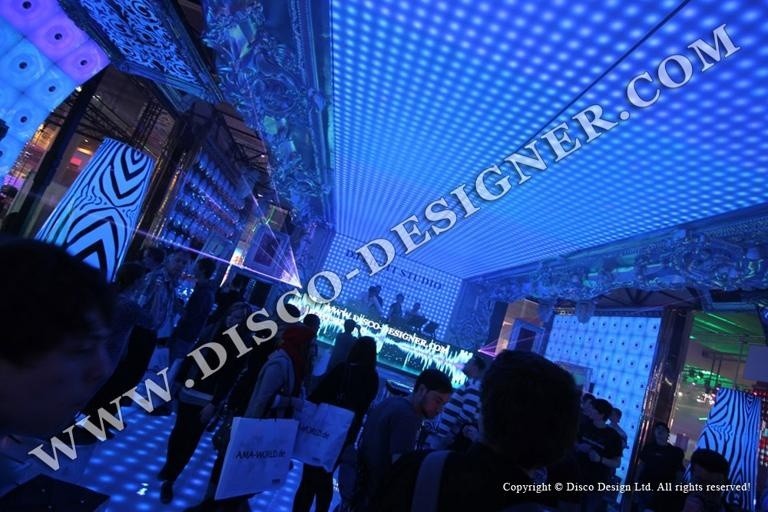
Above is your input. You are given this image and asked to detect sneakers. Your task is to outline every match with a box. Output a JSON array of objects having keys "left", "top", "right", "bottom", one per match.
[{"left": 159, "top": 479, "right": 174, "bottom": 505}]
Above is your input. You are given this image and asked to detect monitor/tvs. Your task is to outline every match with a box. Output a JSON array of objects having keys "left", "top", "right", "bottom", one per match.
[
  {"left": 423, "top": 320, "right": 440, "bottom": 338},
  {"left": 407, "top": 314, "right": 428, "bottom": 333}
]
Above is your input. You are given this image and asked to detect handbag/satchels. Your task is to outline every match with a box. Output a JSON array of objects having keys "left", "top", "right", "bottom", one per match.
[{"left": 213, "top": 359, "right": 301, "bottom": 500}]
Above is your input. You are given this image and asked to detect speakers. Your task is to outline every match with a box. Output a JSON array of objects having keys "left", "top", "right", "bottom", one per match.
[{"left": 265, "top": 206, "right": 288, "bottom": 232}]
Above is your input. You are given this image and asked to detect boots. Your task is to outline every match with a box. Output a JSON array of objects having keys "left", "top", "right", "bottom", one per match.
[{"left": 288, "top": 359, "right": 358, "bottom": 476}]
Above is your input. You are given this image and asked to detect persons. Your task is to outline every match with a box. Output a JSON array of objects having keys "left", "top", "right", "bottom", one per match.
[{"left": 0, "top": 181, "right": 744, "bottom": 512}]
